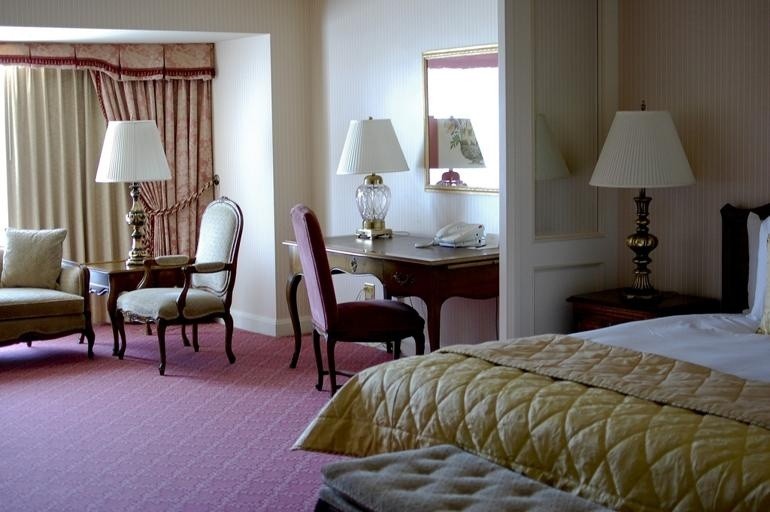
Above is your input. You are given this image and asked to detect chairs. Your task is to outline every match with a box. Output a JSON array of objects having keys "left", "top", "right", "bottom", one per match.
[
  {"left": 116, "top": 197, "right": 243, "bottom": 373},
  {"left": 284, "top": 206, "right": 425, "bottom": 399}
]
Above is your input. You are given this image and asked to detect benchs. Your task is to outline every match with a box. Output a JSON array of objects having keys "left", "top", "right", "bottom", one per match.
[{"left": 310, "top": 440, "right": 609, "bottom": 512}]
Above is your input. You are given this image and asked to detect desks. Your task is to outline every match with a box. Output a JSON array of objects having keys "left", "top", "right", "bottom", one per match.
[{"left": 282, "top": 228, "right": 499, "bottom": 368}]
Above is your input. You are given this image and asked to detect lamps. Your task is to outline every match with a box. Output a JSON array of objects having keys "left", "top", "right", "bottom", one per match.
[
  {"left": 427, "top": 116, "right": 486, "bottom": 188},
  {"left": 336, "top": 116, "right": 409, "bottom": 238},
  {"left": 589, "top": 102, "right": 695, "bottom": 304},
  {"left": 96, "top": 119, "right": 172, "bottom": 265}
]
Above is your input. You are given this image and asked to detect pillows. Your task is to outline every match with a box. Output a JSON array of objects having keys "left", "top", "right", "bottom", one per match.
[
  {"left": 2, "top": 224, "right": 68, "bottom": 291},
  {"left": 745, "top": 212, "right": 760, "bottom": 313},
  {"left": 746, "top": 217, "right": 770, "bottom": 323},
  {"left": 757, "top": 237, "right": 770, "bottom": 334}
]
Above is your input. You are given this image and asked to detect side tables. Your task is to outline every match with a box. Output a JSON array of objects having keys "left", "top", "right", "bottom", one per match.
[{"left": 76, "top": 255, "right": 198, "bottom": 355}]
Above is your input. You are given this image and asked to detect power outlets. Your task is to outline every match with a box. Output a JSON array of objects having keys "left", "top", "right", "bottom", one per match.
[{"left": 362, "top": 282, "right": 376, "bottom": 300}]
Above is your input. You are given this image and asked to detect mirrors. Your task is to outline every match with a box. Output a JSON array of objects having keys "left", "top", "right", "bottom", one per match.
[{"left": 421, "top": 43, "right": 501, "bottom": 194}]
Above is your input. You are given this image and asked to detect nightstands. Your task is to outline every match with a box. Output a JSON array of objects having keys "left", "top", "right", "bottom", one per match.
[{"left": 566, "top": 286, "right": 720, "bottom": 336}]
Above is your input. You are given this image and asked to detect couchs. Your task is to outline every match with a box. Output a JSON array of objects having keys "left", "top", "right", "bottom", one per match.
[{"left": 0, "top": 247, "right": 95, "bottom": 360}]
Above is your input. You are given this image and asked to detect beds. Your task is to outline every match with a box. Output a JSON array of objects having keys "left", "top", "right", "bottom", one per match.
[{"left": 290, "top": 203, "right": 770, "bottom": 512}]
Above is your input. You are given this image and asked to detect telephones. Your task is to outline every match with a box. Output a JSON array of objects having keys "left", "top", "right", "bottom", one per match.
[{"left": 414, "top": 218, "right": 489, "bottom": 252}]
